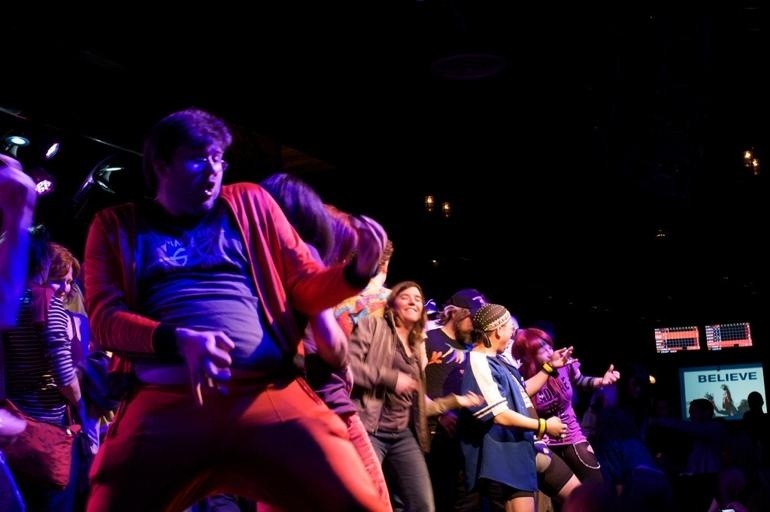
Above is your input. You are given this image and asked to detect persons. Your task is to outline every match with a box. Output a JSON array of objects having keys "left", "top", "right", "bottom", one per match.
[
  {"left": 511, "top": 325, "right": 619, "bottom": 511},
  {"left": 452, "top": 302, "right": 584, "bottom": 512},
  {"left": 671, "top": 399, "right": 726, "bottom": 459},
  {"left": 0, "top": 154, "right": 35, "bottom": 512},
  {"left": 59, "top": 276, "right": 91, "bottom": 383},
  {"left": 744, "top": 392, "right": 769, "bottom": 446},
  {"left": 336, "top": 240, "right": 398, "bottom": 338},
  {"left": 607, "top": 423, "right": 673, "bottom": 497},
  {"left": 345, "top": 280, "right": 483, "bottom": 512},
  {"left": 78, "top": 108, "right": 392, "bottom": 512},
  {"left": 295, "top": 207, "right": 394, "bottom": 512},
  {"left": 422, "top": 290, "right": 489, "bottom": 512},
  {"left": 257, "top": 169, "right": 337, "bottom": 272},
  {"left": 47, "top": 239, "right": 85, "bottom": 369}
]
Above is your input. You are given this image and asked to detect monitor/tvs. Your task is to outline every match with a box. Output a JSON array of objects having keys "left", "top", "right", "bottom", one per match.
[
  {"left": 705, "top": 322, "right": 753, "bottom": 351},
  {"left": 654, "top": 325, "right": 700, "bottom": 356},
  {"left": 681, "top": 364, "right": 770, "bottom": 424}
]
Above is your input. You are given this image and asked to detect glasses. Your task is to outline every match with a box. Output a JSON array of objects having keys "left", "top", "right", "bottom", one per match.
[{"left": 180, "top": 155, "right": 229, "bottom": 174}]
